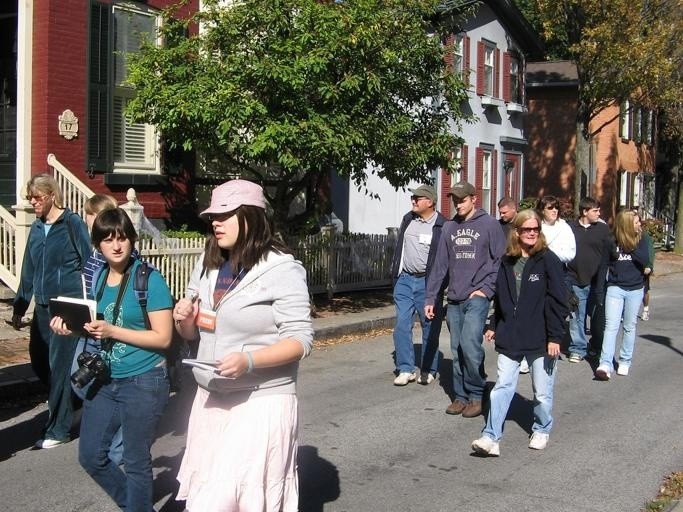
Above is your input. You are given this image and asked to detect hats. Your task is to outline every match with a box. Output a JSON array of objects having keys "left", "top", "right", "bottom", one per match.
[
  {"left": 198, "top": 179, "right": 268, "bottom": 216},
  {"left": 446, "top": 181, "right": 476, "bottom": 199},
  {"left": 407, "top": 184, "right": 437, "bottom": 202}
]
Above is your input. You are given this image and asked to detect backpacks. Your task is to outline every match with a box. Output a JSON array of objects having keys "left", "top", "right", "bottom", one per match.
[{"left": 91, "top": 262, "right": 191, "bottom": 400}]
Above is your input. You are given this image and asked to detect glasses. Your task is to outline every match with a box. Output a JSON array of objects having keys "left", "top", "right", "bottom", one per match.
[
  {"left": 25, "top": 195, "right": 51, "bottom": 201},
  {"left": 544, "top": 206, "right": 560, "bottom": 210},
  {"left": 516, "top": 226, "right": 540, "bottom": 235}
]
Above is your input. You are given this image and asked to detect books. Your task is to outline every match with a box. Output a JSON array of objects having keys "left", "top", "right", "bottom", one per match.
[{"left": 50, "top": 297, "right": 96, "bottom": 333}]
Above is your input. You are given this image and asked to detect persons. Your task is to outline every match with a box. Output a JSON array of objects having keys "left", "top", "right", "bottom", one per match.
[
  {"left": 568, "top": 197, "right": 654, "bottom": 380},
  {"left": 423, "top": 182, "right": 506, "bottom": 417},
  {"left": 171, "top": 181, "right": 314, "bottom": 511},
  {"left": 48, "top": 208, "right": 173, "bottom": 511},
  {"left": 494, "top": 197, "right": 518, "bottom": 241},
  {"left": 519, "top": 195, "right": 577, "bottom": 373},
  {"left": 11, "top": 174, "right": 93, "bottom": 449},
  {"left": 389, "top": 184, "right": 449, "bottom": 384},
  {"left": 470, "top": 208, "right": 567, "bottom": 455},
  {"left": 71, "top": 192, "right": 139, "bottom": 466}
]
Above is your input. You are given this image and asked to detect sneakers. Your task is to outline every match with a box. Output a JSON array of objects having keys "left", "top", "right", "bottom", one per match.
[
  {"left": 471, "top": 435, "right": 500, "bottom": 457},
  {"left": 569, "top": 352, "right": 582, "bottom": 363},
  {"left": 528, "top": 431, "right": 550, "bottom": 450},
  {"left": 519, "top": 359, "right": 530, "bottom": 374},
  {"left": 462, "top": 395, "right": 489, "bottom": 418},
  {"left": 446, "top": 396, "right": 466, "bottom": 415},
  {"left": 394, "top": 370, "right": 417, "bottom": 386},
  {"left": 595, "top": 364, "right": 611, "bottom": 379},
  {"left": 640, "top": 310, "right": 650, "bottom": 321},
  {"left": 417, "top": 371, "right": 440, "bottom": 384},
  {"left": 616, "top": 363, "right": 629, "bottom": 376},
  {"left": 35, "top": 435, "right": 70, "bottom": 449}
]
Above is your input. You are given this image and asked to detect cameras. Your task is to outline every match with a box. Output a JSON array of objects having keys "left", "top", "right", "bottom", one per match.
[{"left": 70, "top": 351, "right": 112, "bottom": 390}]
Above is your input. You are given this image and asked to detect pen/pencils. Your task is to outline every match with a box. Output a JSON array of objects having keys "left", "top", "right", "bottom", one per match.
[{"left": 176, "top": 293, "right": 199, "bottom": 325}]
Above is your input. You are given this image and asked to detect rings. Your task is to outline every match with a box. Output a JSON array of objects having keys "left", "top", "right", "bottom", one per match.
[
  {"left": 554, "top": 352, "right": 557, "bottom": 354},
  {"left": 174, "top": 306, "right": 180, "bottom": 313}
]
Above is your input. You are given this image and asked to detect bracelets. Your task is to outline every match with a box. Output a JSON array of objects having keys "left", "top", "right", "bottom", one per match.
[{"left": 244, "top": 350, "right": 254, "bottom": 375}]
